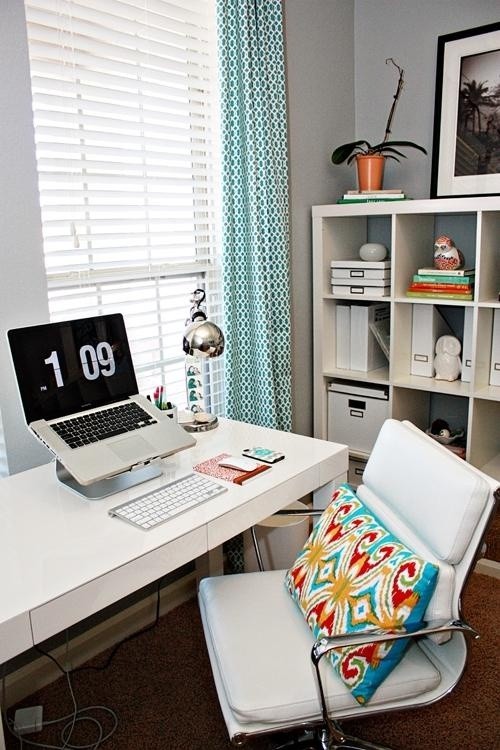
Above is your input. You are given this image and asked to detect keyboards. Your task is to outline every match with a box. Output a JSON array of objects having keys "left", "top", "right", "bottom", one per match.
[{"left": 106, "top": 472, "right": 228, "bottom": 531}]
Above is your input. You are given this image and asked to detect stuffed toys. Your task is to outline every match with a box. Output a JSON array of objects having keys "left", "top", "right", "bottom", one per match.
[
  {"left": 433, "top": 236, "right": 465, "bottom": 269},
  {"left": 434, "top": 334, "right": 462, "bottom": 381},
  {"left": 431, "top": 418, "right": 452, "bottom": 438}
]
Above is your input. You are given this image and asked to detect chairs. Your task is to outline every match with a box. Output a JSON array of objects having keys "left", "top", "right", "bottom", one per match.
[{"left": 196, "top": 418, "right": 500, "bottom": 750}]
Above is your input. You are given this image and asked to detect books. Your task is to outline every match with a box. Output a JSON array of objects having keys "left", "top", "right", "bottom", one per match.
[
  {"left": 337, "top": 189, "right": 415, "bottom": 204},
  {"left": 192, "top": 453, "right": 272, "bottom": 486},
  {"left": 405, "top": 267, "right": 475, "bottom": 302}
]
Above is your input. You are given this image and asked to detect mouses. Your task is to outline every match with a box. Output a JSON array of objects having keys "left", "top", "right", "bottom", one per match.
[{"left": 217, "top": 455, "right": 260, "bottom": 472}]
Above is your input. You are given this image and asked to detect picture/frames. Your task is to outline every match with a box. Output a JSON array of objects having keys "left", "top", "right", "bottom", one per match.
[{"left": 430, "top": 21, "right": 500, "bottom": 199}]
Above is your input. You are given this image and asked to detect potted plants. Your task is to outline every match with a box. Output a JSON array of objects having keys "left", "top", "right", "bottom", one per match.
[{"left": 331, "top": 139, "right": 428, "bottom": 194}]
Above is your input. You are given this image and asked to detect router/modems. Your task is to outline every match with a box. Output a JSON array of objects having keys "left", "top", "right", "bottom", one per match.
[{"left": 15, "top": 706, "right": 44, "bottom": 738}]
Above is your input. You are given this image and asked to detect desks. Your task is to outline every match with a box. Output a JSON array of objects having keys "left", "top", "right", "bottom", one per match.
[{"left": 0, "top": 409, "right": 350, "bottom": 666}]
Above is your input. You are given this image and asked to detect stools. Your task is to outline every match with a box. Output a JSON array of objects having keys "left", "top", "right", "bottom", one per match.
[{"left": 243, "top": 500, "right": 310, "bottom": 574}]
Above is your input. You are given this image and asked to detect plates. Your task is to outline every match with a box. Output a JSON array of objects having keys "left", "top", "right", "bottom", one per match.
[{"left": 426, "top": 426, "right": 464, "bottom": 445}]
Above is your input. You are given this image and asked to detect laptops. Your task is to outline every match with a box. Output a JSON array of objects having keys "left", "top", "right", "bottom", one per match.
[{"left": 6, "top": 312, "right": 197, "bottom": 485}]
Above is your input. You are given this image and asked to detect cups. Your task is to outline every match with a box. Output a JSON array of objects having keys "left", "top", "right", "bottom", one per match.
[{"left": 160, "top": 403, "right": 178, "bottom": 424}]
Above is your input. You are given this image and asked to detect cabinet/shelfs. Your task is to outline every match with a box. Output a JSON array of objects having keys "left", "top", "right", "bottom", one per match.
[{"left": 311, "top": 196, "right": 500, "bottom": 580}]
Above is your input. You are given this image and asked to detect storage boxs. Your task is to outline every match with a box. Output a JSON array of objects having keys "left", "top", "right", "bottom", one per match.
[{"left": 326, "top": 380, "right": 390, "bottom": 454}]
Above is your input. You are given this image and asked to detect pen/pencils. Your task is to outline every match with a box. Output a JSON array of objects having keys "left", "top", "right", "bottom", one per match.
[{"left": 153, "top": 385, "right": 163, "bottom": 411}]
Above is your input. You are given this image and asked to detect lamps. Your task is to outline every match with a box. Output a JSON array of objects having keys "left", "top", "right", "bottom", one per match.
[{"left": 179, "top": 287, "right": 226, "bottom": 433}]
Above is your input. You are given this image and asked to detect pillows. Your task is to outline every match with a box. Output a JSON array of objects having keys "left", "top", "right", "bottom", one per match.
[{"left": 282, "top": 486, "right": 441, "bottom": 707}]
